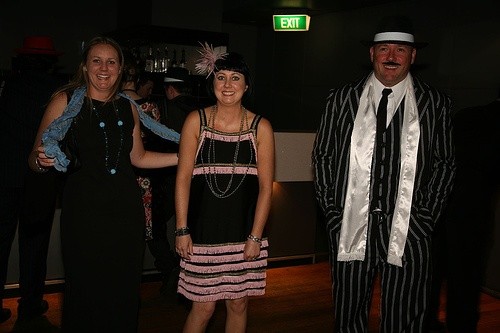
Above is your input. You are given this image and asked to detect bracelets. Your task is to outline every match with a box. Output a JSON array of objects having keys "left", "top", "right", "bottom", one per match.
[
  {"left": 175, "top": 228, "right": 190, "bottom": 236},
  {"left": 35, "top": 160, "right": 45, "bottom": 172},
  {"left": 249, "top": 234, "right": 261, "bottom": 242}
]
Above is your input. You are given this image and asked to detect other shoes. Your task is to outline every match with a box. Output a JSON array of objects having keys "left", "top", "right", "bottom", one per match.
[
  {"left": 0, "top": 309, "right": 10, "bottom": 323},
  {"left": 18, "top": 300, "right": 48, "bottom": 320}
]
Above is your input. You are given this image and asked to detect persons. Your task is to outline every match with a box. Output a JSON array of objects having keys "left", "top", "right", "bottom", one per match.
[
  {"left": 29, "top": 37, "right": 179, "bottom": 333},
  {"left": 173, "top": 53, "right": 275, "bottom": 333},
  {"left": 423, "top": 90, "right": 500, "bottom": 332},
  {"left": 0, "top": 52, "right": 59, "bottom": 321},
  {"left": 312, "top": 24, "right": 455, "bottom": 333},
  {"left": 119, "top": 51, "right": 201, "bottom": 293}
]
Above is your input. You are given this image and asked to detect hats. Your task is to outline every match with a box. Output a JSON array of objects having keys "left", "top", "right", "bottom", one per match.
[
  {"left": 371, "top": 16, "right": 416, "bottom": 48},
  {"left": 160, "top": 67, "right": 192, "bottom": 87},
  {"left": 18, "top": 35, "right": 64, "bottom": 56}
]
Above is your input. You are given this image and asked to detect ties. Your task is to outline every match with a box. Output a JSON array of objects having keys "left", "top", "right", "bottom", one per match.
[{"left": 376, "top": 88, "right": 392, "bottom": 143}]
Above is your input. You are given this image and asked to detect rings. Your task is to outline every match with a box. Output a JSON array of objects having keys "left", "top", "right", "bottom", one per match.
[
  {"left": 179, "top": 253, "right": 181, "bottom": 255},
  {"left": 253, "top": 258, "right": 256, "bottom": 260}
]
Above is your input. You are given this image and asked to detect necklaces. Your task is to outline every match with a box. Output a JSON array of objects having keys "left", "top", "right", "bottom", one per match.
[
  {"left": 201, "top": 104, "right": 252, "bottom": 198},
  {"left": 92, "top": 99, "right": 124, "bottom": 174}
]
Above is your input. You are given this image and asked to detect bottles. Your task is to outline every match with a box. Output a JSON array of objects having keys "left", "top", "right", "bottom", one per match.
[{"left": 132, "top": 45, "right": 187, "bottom": 75}]
